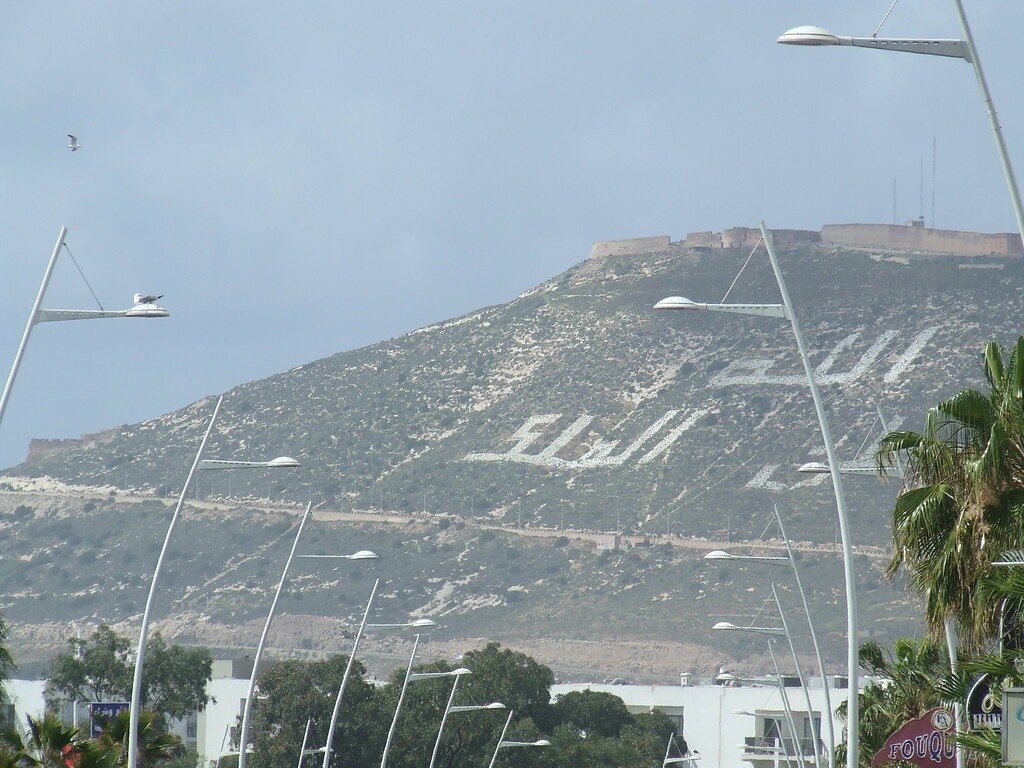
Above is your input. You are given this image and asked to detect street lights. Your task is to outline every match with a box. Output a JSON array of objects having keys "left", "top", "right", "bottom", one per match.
[
  {"left": 777, "top": 3, "right": 1022, "bottom": 252},
  {"left": 799, "top": 397, "right": 969, "bottom": 768},
  {"left": 2, "top": 224, "right": 172, "bottom": 425},
  {"left": 236, "top": 500, "right": 551, "bottom": 767},
  {"left": 650, "top": 222, "right": 858, "bottom": 768},
  {"left": 123, "top": 397, "right": 303, "bottom": 768}
]
